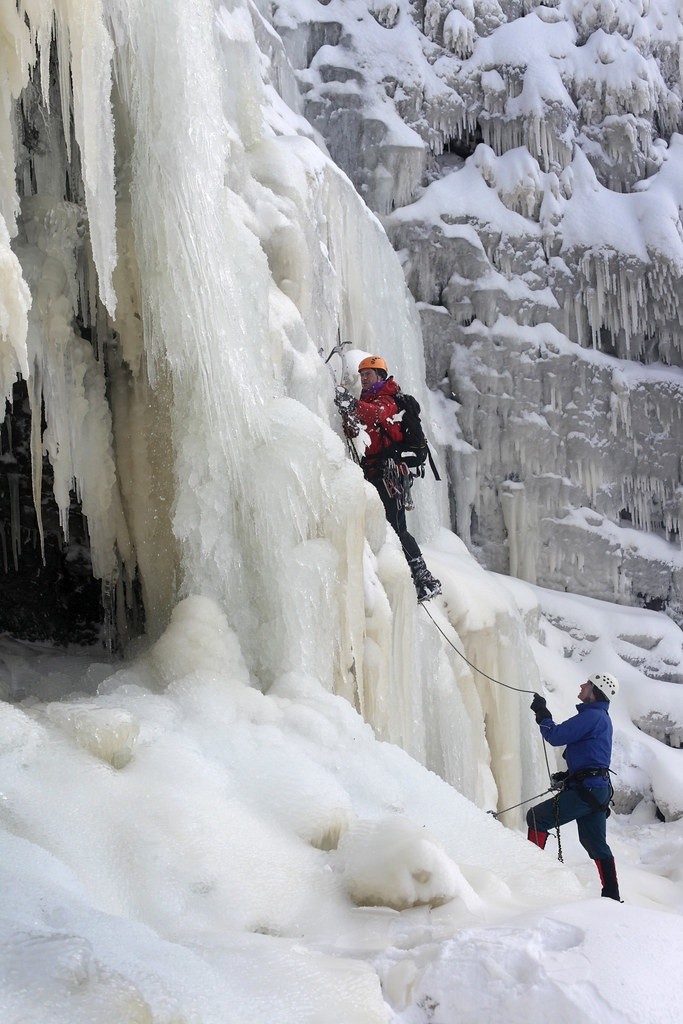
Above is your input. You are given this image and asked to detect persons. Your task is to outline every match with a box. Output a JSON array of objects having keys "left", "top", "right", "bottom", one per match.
[
  {"left": 527, "top": 671, "right": 622, "bottom": 905},
  {"left": 337, "top": 356, "right": 441, "bottom": 601}
]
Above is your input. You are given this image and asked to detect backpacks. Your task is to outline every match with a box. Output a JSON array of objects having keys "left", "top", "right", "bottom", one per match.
[{"left": 369, "top": 392, "right": 427, "bottom": 468}]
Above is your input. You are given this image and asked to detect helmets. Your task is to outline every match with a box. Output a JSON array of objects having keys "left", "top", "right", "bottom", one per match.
[
  {"left": 358, "top": 356, "right": 388, "bottom": 379},
  {"left": 587, "top": 673, "right": 619, "bottom": 702}
]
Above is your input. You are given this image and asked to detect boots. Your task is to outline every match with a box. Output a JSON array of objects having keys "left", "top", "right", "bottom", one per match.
[
  {"left": 407, "top": 556, "right": 441, "bottom": 599},
  {"left": 527, "top": 828, "right": 555, "bottom": 851},
  {"left": 594, "top": 856, "right": 624, "bottom": 903}
]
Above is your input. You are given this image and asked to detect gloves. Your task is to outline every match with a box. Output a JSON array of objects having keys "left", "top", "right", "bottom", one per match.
[
  {"left": 333, "top": 390, "right": 358, "bottom": 414},
  {"left": 551, "top": 769, "right": 569, "bottom": 783},
  {"left": 530, "top": 693, "right": 552, "bottom": 724}
]
